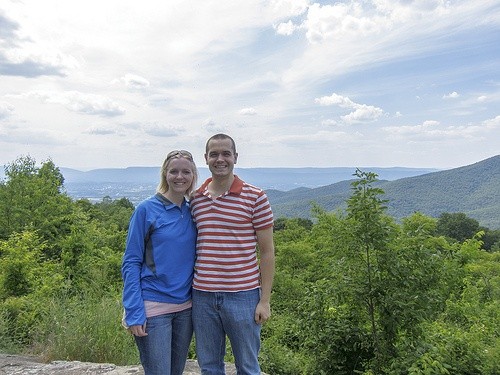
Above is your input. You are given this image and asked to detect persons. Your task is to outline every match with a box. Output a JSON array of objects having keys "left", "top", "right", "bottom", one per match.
[
  {"left": 190, "top": 134, "right": 274, "bottom": 375},
  {"left": 122, "top": 151, "right": 198, "bottom": 375}
]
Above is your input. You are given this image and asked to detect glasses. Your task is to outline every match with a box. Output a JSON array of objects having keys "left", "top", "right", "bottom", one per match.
[{"left": 167, "top": 150, "right": 192, "bottom": 160}]
